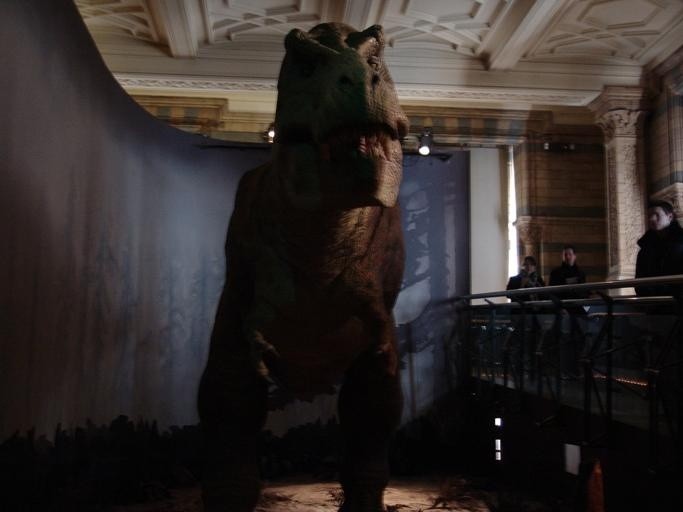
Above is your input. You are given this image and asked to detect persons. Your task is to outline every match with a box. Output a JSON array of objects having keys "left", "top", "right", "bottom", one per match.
[
  {"left": 633, "top": 198, "right": 682, "bottom": 299},
  {"left": 549, "top": 246, "right": 590, "bottom": 300},
  {"left": 505, "top": 255, "right": 548, "bottom": 301}
]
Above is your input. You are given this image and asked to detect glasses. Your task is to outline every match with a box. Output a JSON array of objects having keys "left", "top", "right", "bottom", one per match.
[
  {"left": 417, "top": 127, "right": 433, "bottom": 156},
  {"left": 268, "top": 126, "right": 275, "bottom": 138}
]
